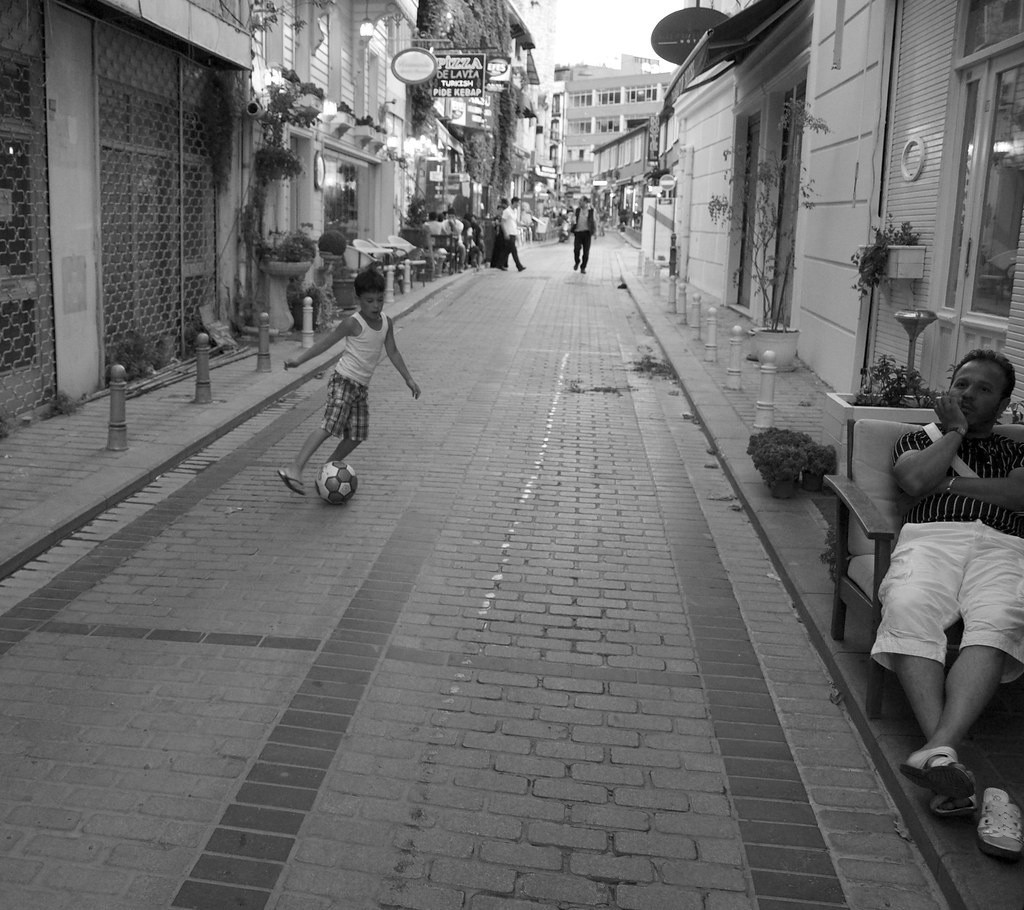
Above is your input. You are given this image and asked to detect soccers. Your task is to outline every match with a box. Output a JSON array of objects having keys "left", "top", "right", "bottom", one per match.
[{"left": 315, "top": 459, "right": 359, "bottom": 504}]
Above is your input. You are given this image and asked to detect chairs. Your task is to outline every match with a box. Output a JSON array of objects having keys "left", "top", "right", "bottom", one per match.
[
  {"left": 833, "top": 417, "right": 1024, "bottom": 726},
  {"left": 431, "top": 234, "right": 463, "bottom": 275},
  {"left": 344, "top": 234, "right": 428, "bottom": 295}
]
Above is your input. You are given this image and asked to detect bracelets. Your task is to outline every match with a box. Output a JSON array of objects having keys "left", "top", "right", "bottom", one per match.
[
  {"left": 946, "top": 476, "right": 958, "bottom": 494},
  {"left": 943, "top": 426, "right": 967, "bottom": 436}
]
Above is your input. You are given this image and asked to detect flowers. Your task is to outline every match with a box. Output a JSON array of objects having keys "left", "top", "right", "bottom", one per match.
[
  {"left": 356, "top": 114, "right": 375, "bottom": 127},
  {"left": 337, "top": 100, "right": 356, "bottom": 117},
  {"left": 375, "top": 125, "right": 387, "bottom": 133},
  {"left": 300, "top": 81, "right": 326, "bottom": 101}
]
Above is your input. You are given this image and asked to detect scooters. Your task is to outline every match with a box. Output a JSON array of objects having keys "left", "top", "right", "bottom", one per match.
[{"left": 559, "top": 217, "right": 571, "bottom": 242}]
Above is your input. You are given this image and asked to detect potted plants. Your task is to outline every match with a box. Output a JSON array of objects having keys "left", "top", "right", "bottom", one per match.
[
  {"left": 252, "top": 143, "right": 307, "bottom": 187},
  {"left": 286, "top": 275, "right": 323, "bottom": 331},
  {"left": 745, "top": 426, "right": 838, "bottom": 501},
  {"left": 823, "top": 352, "right": 1024, "bottom": 474},
  {"left": 847, "top": 212, "right": 927, "bottom": 302},
  {"left": 260, "top": 222, "right": 316, "bottom": 278},
  {"left": 393, "top": 194, "right": 432, "bottom": 249},
  {"left": 708, "top": 96, "right": 831, "bottom": 373},
  {"left": 317, "top": 231, "right": 348, "bottom": 270}
]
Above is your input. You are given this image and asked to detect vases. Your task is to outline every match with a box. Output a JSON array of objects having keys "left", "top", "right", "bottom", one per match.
[
  {"left": 333, "top": 111, "right": 356, "bottom": 132},
  {"left": 354, "top": 125, "right": 376, "bottom": 142},
  {"left": 331, "top": 278, "right": 358, "bottom": 310},
  {"left": 368, "top": 133, "right": 388, "bottom": 146},
  {"left": 295, "top": 94, "right": 323, "bottom": 116}
]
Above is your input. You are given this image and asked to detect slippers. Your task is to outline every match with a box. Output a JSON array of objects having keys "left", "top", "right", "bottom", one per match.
[
  {"left": 977, "top": 786, "right": 1024, "bottom": 859},
  {"left": 931, "top": 790, "right": 976, "bottom": 817},
  {"left": 898, "top": 746, "right": 974, "bottom": 799},
  {"left": 277, "top": 469, "right": 306, "bottom": 495}
]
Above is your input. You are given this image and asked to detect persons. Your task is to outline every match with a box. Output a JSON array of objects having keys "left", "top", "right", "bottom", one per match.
[
  {"left": 491, "top": 198, "right": 507, "bottom": 274},
  {"left": 569, "top": 196, "right": 597, "bottom": 274},
  {"left": 278, "top": 269, "right": 420, "bottom": 495},
  {"left": 870, "top": 348, "right": 1024, "bottom": 816},
  {"left": 423, "top": 205, "right": 485, "bottom": 281},
  {"left": 502, "top": 197, "right": 527, "bottom": 272},
  {"left": 557, "top": 205, "right": 608, "bottom": 242}
]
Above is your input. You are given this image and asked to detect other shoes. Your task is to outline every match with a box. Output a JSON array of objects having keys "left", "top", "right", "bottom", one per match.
[
  {"left": 573, "top": 264, "right": 578, "bottom": 270},
  {"left": 518, "top": 267, "right": 526, "bottom": 272},
  {"left": 581, "top": 265, "right": 586, "bottom": 274}
]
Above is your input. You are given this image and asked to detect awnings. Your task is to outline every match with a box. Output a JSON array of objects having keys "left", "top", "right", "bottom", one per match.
[{"left": 664, "top": 0, "right": 791, "bottom": 107}]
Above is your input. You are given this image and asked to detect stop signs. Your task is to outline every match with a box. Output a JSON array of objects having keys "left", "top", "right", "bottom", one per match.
[{"left": 659, "top": 174, "right": 675, "bottom": 191}]
